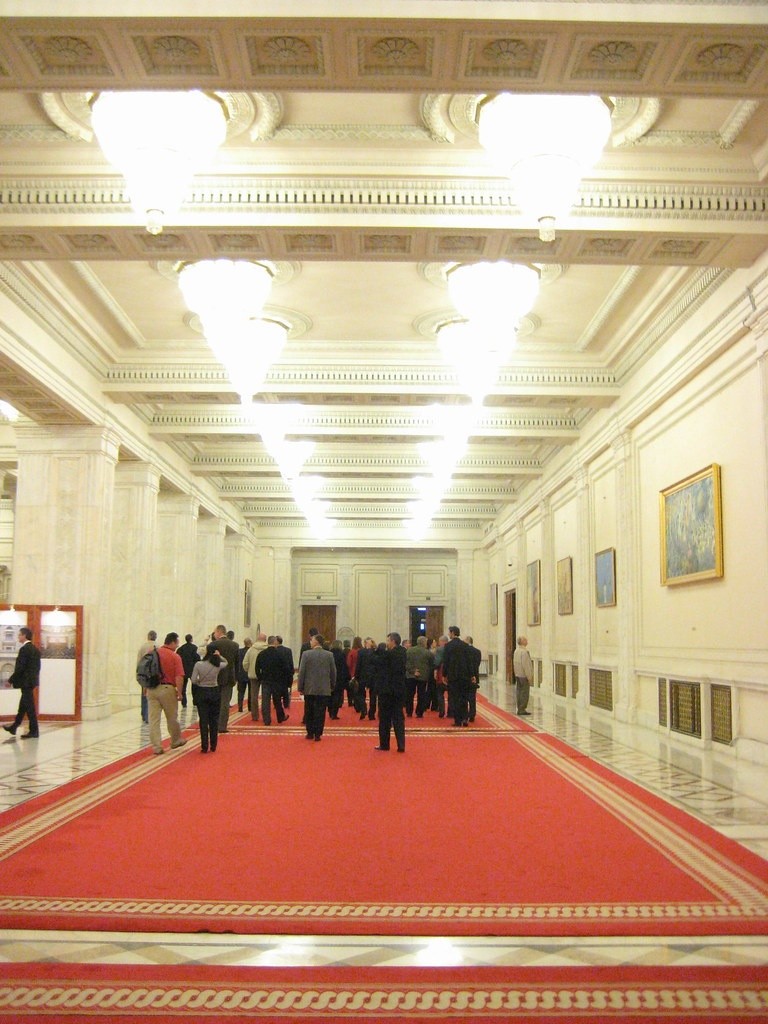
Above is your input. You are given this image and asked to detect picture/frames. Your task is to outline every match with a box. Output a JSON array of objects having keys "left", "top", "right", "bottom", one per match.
[
  {"left": 557, "top": 557, "right": 572, "bottom": 613},
  {"left": 658, "top": 462, "right": 723, "bottom": 586},
  {"left": 526, "top": 560, "right": 542, "bottom": 626},
  {"left": 490, "top": 584, "right": 498, "bottom": 625},
  {"left": 597, "top": 547, "right": 617, "bottom": 607}
]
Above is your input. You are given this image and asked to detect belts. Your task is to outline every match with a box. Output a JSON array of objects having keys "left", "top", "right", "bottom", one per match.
[{"left": 159, "top": 683, "right": 171, "bottom": 685}]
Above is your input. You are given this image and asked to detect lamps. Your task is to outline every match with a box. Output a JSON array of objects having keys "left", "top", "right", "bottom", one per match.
[
  {"left": 97, "top": 93, "right": 292, "bottom": 412},
  {"left": 433, "top": 88, "right": 615, "bottom": 412}
]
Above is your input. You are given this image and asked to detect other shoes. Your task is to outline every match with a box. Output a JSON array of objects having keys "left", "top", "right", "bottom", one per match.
[
  {"left": 21, "top": 732, "right": 39, "bottom": 738},
  {"left": 278, "top": 715, "right": 289, "bottom": 722},
  {"left": 154, "top": 750, "right": 163, "bottom": 754},
  {"left": 517, "top": 712, "right": 531, "bottom": 715},
  {"left": 171, "top": 739, "right": 186, "bottom": 749},
  {"left": 374, "top": 746, "right": 389, "bottom": 750},
  {"left": 330, "top": 702, "right": 474, "bottom": 726},
  {"left": 306, "top": 733, "right": 313, "bottom": 738},
  {"left": 397, "top": 748, "right": 404, "bottom": 752},
  {"left": 211, "top": 747, "right": 215, "bottom": 752},
  {"left": 201, "top": 750, "right": 207, "bottom": 753},
  {"left": 2, "top": 725, "right": 16, "bottom": 735},
  {"left": 316, "top": 737, "right": 321, "bottom": 740}
]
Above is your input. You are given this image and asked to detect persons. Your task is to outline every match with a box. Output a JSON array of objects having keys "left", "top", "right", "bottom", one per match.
[
  {"left": 136, "top": 630, "right": 158, "bottom": 722},
  {"left": 176, "top": 633, "right": 200, "bottom": 707},
  {"left": 190, "top": 642, "right": 229, "bottom": 753},
  {"left": 2, "top": 629, "right": 41, "bottom": 738},
  {"left": 208, "top": 624, "right": 295, "bottom": 732},
  {"left": 300, "top": 628, "right": 452, "bottom": 722},
  {"left": 512, "top": 636, "right": 533, "bottom": 715},
  {"left": 441, "top": 626, "right": 477, "bottom": 727},
  {"left": 464, "top": 635, "right": 481, "bottom": 722},
  {"left": 143, "top": 632, "right": 186, "bottom": 754},
  {"left": 298, "top": 634, "right": 337, "bottom": 741},
  {"left": 368, "top": 632, "right": 406, "bottom": 752}
]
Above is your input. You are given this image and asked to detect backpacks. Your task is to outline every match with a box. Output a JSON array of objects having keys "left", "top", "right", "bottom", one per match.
[{"left": 136, "top": 649, "right": 164, "bottom": 688}]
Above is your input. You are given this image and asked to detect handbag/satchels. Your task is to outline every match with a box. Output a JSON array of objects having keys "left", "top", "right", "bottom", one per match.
[{"left": 193, "top": 687, "right": 204, "bottom": 705}]
